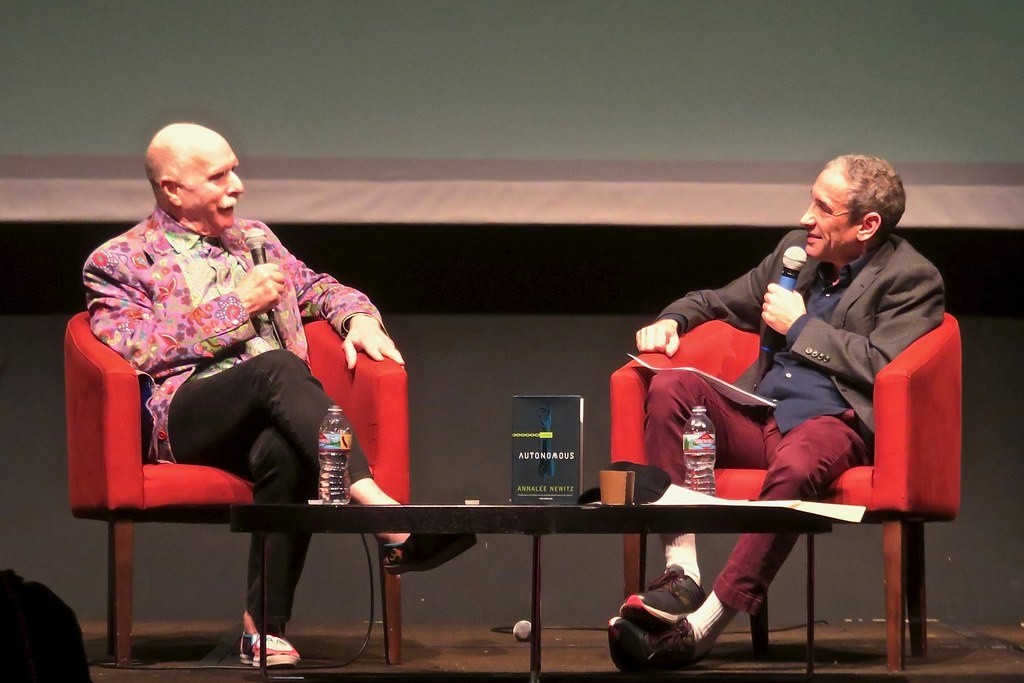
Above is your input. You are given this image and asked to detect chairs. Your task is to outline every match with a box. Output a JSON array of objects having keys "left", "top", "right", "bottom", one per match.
[
  {"left": 609, "top": 309, "right": 964, "bottom": 672},
  {"left": 64, "top": 308, "right": 408, "bottom": 668}
]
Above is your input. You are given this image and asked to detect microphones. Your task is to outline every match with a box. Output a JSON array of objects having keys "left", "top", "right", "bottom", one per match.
[
  {"left": 761, "top": 246, "right": 808, "bottom": 353},
  {"left": 512, "top": 620, "right": 531, "bottom": 642},
  {"left": 245, "top": 227, "right": 276, "bottom": 322}
]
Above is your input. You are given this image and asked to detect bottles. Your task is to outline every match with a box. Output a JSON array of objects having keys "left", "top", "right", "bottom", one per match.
[
  {"left": 318, "top": 404, "right": 353, "bottom": 506},
  {"left": 682, "top": 405, "right": 717, "bottom": 497}
]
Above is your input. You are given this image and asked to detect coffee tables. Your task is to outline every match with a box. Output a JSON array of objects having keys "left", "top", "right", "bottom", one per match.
[{"left": 229, "top": 503, "right": 832, "bottom": 679}]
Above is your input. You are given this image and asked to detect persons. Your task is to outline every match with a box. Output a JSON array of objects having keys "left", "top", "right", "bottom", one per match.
[
  {"left": 608, "top": 155, "right": 944, "bottom": 671},
  {"left": 84, "top": 124, "right": 476, "bottom": 667}
]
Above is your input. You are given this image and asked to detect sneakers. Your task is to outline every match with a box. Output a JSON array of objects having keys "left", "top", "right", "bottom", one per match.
[
  {"left": 607, "top": 617, "right": 711, "bottom": 674},
  {"left": 620, "top": 564, "right": 706, "bottom": 634},
  {"left": 383, "top": 532, "right": 477, "bottom": 575},
  {"left": 239, "top": 631, "right": 301, "bottom": 668}
]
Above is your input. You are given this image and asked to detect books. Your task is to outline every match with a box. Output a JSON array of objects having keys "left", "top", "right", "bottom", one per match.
[{"left": 512, "top": 395, "right": 584, "bottom": 504}]
